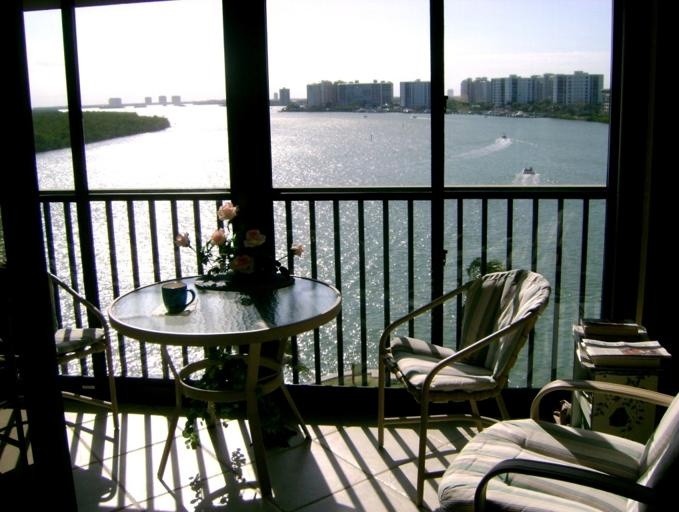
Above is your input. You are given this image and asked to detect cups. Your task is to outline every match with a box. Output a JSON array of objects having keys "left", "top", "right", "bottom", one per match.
[{"left": 162, "top": 282, "right": 196, "bottom": 314}]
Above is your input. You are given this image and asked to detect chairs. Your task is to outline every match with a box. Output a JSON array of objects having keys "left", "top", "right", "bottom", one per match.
[
  {"left": 377, "top": 269, "right": 551, "bottom": 506},
  {"left": 437, "top": 377, "right": 679, "bottom": 512},
  {"left": 46, "top": 270, "right": 119, "bottom": 430}
]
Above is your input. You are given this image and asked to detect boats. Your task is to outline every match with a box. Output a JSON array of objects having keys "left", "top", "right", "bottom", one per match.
[
  {"left": 500, "top": 133, "right": 507, "bottom": 139},
  {"left": 522, "top": 166, "right": 536, "bottom": 177}
]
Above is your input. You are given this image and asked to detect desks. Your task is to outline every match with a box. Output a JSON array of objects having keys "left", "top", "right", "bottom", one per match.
[{"left": 107, "top": 274, "right": 342, "bottom": 497}]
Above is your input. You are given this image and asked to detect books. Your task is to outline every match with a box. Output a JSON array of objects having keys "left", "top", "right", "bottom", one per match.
[{"left": 571, "top": 323, "right": 671, "bottom": 367}]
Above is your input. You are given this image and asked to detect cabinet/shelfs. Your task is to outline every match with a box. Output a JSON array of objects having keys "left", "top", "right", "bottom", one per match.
[{"left": 570, "top": 335, "right": 659, "bottom": 446}]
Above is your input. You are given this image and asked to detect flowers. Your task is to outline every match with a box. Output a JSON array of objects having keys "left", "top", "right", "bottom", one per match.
[{"left": 176, "top": 201, "right": 304, "bottom": 277}]
